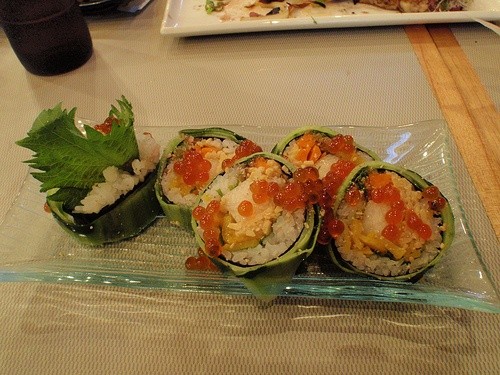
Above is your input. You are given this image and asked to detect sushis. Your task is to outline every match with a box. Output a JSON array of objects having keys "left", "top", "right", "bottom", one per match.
[{"left": 46, "top": 126, "right": 456, "bottom": 301}]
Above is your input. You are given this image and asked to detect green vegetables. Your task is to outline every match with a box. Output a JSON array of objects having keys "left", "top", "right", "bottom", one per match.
[{"left": 14, "top": 95, "right": 141, "bottom": 195}]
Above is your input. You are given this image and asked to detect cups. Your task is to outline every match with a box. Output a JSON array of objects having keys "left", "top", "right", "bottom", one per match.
[{"left": 0, "top": 0, "right": 93, "bottom": 76}]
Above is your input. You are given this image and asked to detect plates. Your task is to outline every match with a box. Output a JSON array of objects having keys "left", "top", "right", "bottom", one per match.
[
  {"left": 160, "top": 0, "right": 499, "bottom": 38},
  {"left": 0, "top": 118, "right": 499, "bottom": 313}
]
